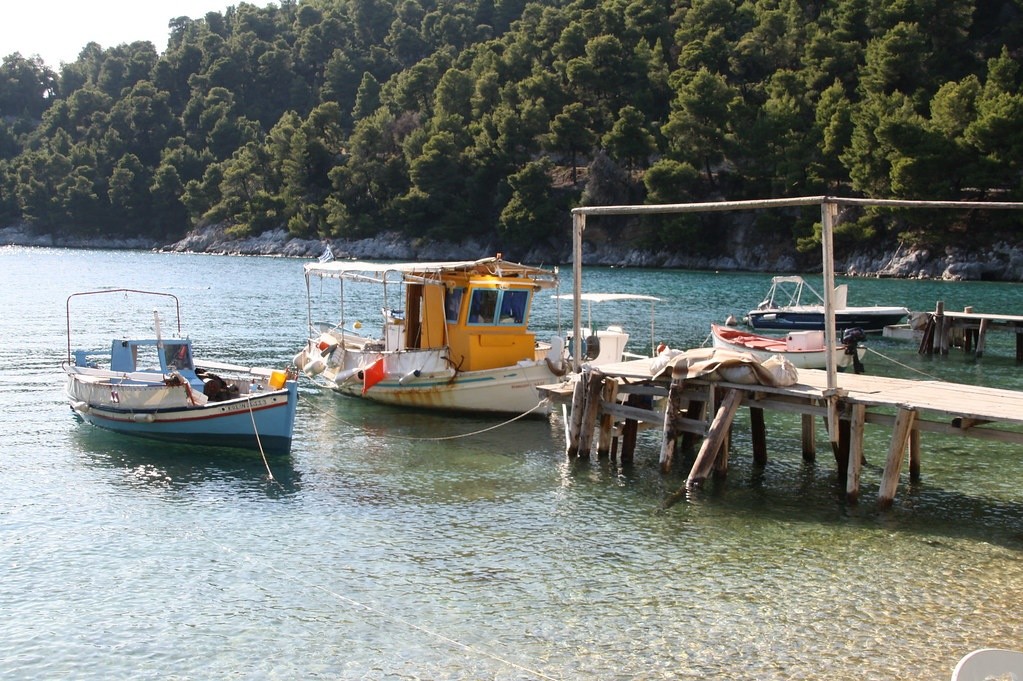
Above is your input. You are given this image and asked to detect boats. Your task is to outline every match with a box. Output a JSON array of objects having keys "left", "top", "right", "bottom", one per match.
[
  {"left": 741, "top": 275, "right": 913, "bottom": 333},
  {"left": 708, "top": 322, "right": 868, "bottom": 369},
  {"left": 67, "top": 288, "right": 300, "bottom": 458},
  {"left": 544, "top": 291, "right": 671, "bottom": 408},
  {"left": 292, "top": 242, "right": 565, "bottom": 418}
]
